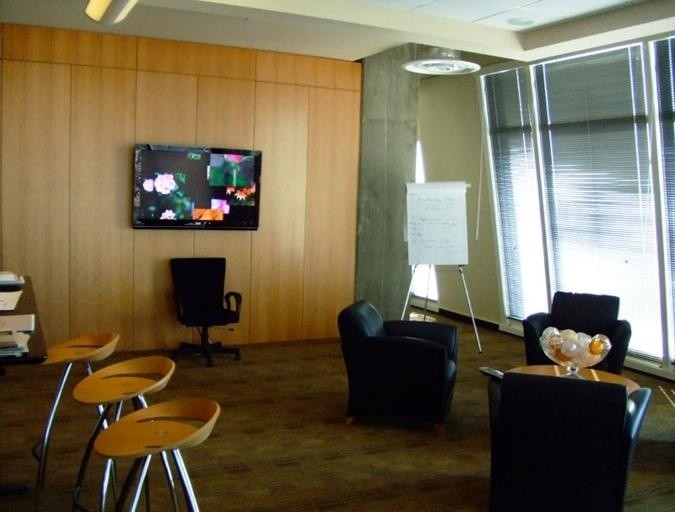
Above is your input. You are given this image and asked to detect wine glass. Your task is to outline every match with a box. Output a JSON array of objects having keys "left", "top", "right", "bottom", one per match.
[{"left": 539, "top": 332, "right": 612, "bottom": 380}]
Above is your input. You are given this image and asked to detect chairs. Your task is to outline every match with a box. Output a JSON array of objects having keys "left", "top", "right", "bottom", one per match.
[
  {"left": 168, "top": 256, "right": 248, "bottom": 367},
  {"left": 488, "top": 371, "right": 651, "bottom": 512},
  {"left": 338, "top": 299, "right": 460, "bottom": 438},
  {"left": 523, "top": 292, "right": 633, "bottom": 373}
]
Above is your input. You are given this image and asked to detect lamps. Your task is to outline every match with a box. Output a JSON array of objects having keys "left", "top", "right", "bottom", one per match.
[{"left": 401, "top": 45, "right": 481, "bottom": 76}]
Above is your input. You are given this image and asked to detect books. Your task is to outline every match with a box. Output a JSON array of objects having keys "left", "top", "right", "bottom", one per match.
[{"left": 0, "top": 270, "right": 37, "bottom": 359}]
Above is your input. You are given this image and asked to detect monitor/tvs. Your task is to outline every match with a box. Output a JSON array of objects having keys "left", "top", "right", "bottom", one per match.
[{"left": 133, "top": 143, "right": 264, "bottom": 231}]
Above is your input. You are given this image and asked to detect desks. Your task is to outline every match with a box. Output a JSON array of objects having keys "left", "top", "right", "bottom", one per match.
[
  {"left": 0, "top": 274, "right": 48, "bottom": 367},
  {"left": 506, "top": 363, "right": 641, "bottom": 396}
]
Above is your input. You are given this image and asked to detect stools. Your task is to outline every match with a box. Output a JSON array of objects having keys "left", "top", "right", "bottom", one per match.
[{"left": 30, "top": 330, "right": 220, "bottom": 511}]
